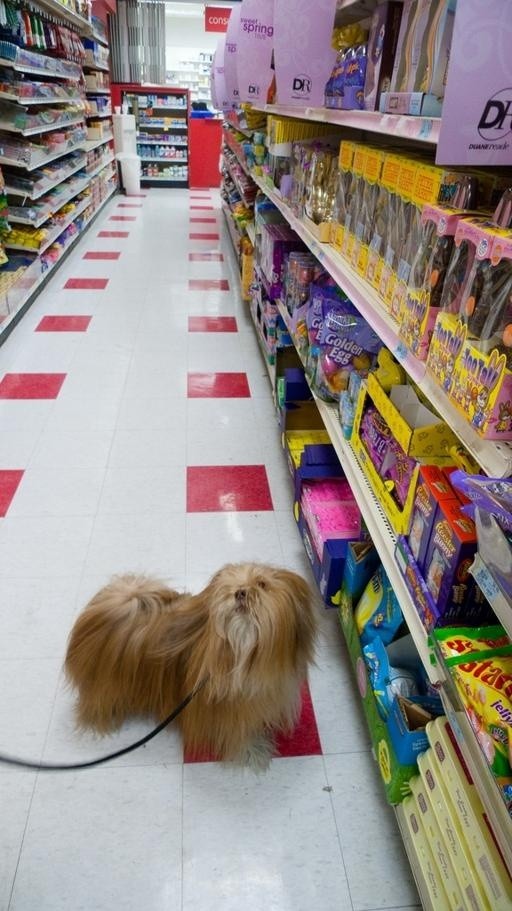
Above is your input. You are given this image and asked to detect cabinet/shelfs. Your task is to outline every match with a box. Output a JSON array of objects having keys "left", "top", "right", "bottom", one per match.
[
  {"left": 179, "top": 51, "right": 213, "bottom": 112},
  {"left": 0, "top": 0, "right": 119, "bottom": 347},
  {"left": 112, "top": 84, "right": 189, "bottom": 188},
  {"left": 218, "top": 0, "right": 512, "bottom": 911}
]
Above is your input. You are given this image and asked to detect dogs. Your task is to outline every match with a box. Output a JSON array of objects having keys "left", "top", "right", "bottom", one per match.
[{"left": 60, "top": 561, "right": 323, "bottom": 777}]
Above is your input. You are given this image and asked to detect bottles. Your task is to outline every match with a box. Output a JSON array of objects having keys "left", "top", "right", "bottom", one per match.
[{"left": 138, "top": 144, "right": 188, "bottom": 177}]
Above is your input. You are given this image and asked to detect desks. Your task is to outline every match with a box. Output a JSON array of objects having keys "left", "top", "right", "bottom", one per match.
[{"left": 188, "top": 116, "right": 224, "bottom": 189}]
